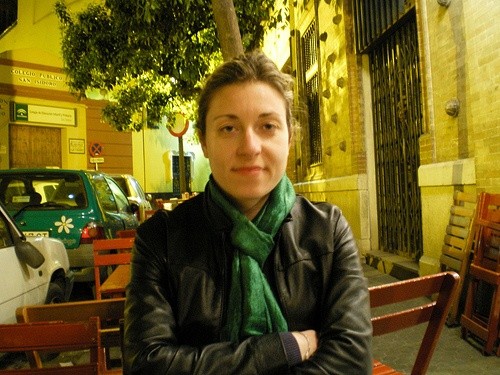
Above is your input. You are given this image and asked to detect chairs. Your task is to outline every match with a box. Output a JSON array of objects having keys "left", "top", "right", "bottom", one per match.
[
  {"left": 437, "top": 189, "right": 500, "bottom": 357},
  {"left": 368, "top": 270, "right": 463, "bottom": 375},
  {"left": 0, "top": 228, "right": 140, "bottom": 375}
]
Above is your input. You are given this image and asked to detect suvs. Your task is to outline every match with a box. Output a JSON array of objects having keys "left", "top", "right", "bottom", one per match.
[{"left": 0, "top": 170, "right": 137, "bottom": 282}]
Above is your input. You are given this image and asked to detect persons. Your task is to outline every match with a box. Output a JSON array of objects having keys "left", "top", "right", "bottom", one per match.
[{"left": 123, "top": 46, "right": 376, "bottom": 375}]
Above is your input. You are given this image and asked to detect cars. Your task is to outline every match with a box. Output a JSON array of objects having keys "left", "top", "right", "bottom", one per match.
[
  {"left": 0, "top": 205, "right": 73, "bottom": 324},
  {"left": 112, "top": 174, "right": 152, "bottom": 226}
]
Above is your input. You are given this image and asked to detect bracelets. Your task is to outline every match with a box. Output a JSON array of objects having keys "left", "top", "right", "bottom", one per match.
[{"left": 299, "top": 331, "right": 312, "bottom": 359}]
